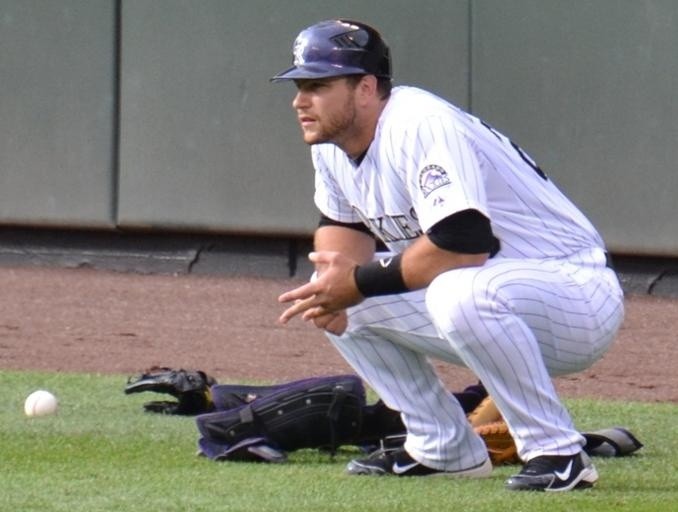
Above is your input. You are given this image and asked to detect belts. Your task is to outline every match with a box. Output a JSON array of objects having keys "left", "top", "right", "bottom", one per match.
[{"left": 605, "top": 251, "right": 619, "bottom": 279}]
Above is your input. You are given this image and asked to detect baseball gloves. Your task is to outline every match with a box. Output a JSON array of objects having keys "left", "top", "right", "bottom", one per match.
[
  {"left": 125, "top": 365, "right": 219, "bottom": 415},
  {"left": 465, "top": 397, "right": 522, "bottom": 466}
]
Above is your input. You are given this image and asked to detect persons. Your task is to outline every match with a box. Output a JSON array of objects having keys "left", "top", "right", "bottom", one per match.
[{"left": 266, "top": 14, "right": 625, "bottom": 493}]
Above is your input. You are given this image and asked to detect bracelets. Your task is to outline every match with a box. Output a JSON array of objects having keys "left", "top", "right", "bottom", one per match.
[{"left": 353, "top": 253, "right": 411, "bottom": 298}]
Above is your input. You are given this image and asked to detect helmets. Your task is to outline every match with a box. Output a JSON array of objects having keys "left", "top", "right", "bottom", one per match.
[{"left": 266, "top": 20, "right": 394, "bottom": 82}]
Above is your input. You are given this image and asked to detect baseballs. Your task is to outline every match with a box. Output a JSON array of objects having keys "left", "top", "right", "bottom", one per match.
[{"left": 24, "top": 390, "right": 57, "bottom": 417}]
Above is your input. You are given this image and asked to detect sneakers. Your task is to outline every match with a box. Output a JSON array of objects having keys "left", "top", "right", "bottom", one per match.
[
  {"left": 347, "top": 443, "right": 492, "bottom": 478},
  {"left": 504, "top": 448, "right": 599, "bottom": 493}
]
